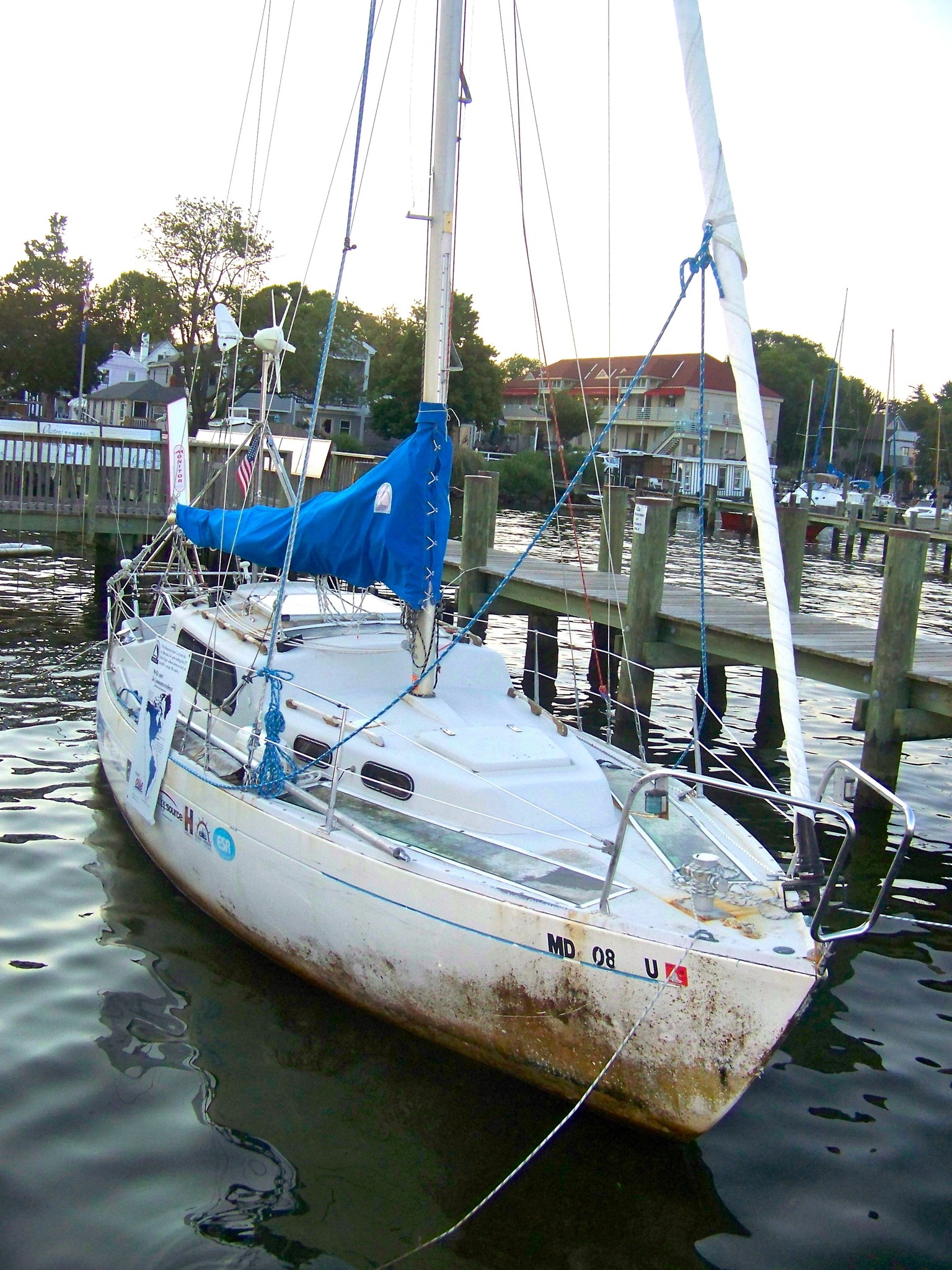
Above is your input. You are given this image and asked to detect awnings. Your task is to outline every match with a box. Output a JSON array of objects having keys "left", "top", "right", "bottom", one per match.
[
  {"left": 501, "top": 388, "right": 539, "bottom": 397},
  {"left": 644, "top": 388, "right": 686, "bottom": 397},
  {"left": 566, "top": 387, "right": 621, "bottom": 396}
]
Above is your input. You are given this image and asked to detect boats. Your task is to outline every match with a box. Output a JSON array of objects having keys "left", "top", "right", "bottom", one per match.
[
  {"left": 89, "top": 0, "right": 922, "bottom": 1143},
  {"left": 775, "top": 287, "right": 952, "bottom": 533}
]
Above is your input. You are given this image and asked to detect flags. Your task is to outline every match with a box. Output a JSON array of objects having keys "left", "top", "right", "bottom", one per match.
[{"left": 235, "top": 429, "right": 259, "bottom": 499}]
